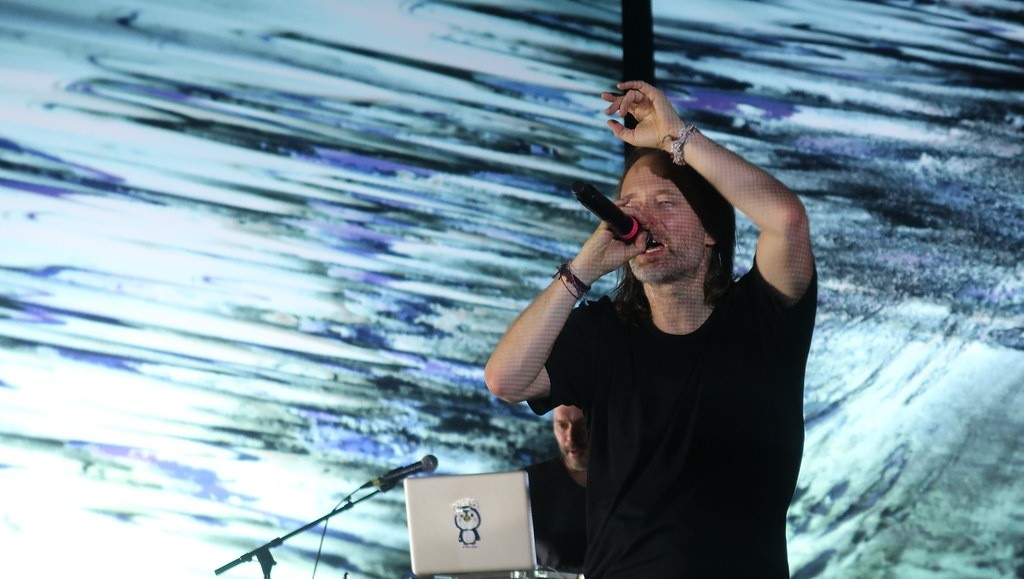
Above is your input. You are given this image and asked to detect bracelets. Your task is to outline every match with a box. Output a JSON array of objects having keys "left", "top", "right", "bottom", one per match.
[
  {"left": 671, "top": 123, "right": 698, "bottom": 167},
  {"left": 552, "top": 260, "right": 591, "bottom": 302}
]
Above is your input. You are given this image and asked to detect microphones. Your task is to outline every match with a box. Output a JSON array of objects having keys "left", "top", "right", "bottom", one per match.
[
  {"left": 571, "top": 181, "right": 653, "bottom": 248},
  {"left": 359, "top": 455, "right": 438, "bottom": 489}
]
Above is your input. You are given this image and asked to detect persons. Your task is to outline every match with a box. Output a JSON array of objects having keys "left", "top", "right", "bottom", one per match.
[
  {"left": 482, "top": 81, "right": 818, "bottom": 579},
  {"left": 516, "top": 403, "right": 589, "bottom": 573}
]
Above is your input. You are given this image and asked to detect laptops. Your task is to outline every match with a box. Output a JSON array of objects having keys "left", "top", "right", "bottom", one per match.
[{"left": 404, "top": 472, "right": 586, "bottom": 579}]
